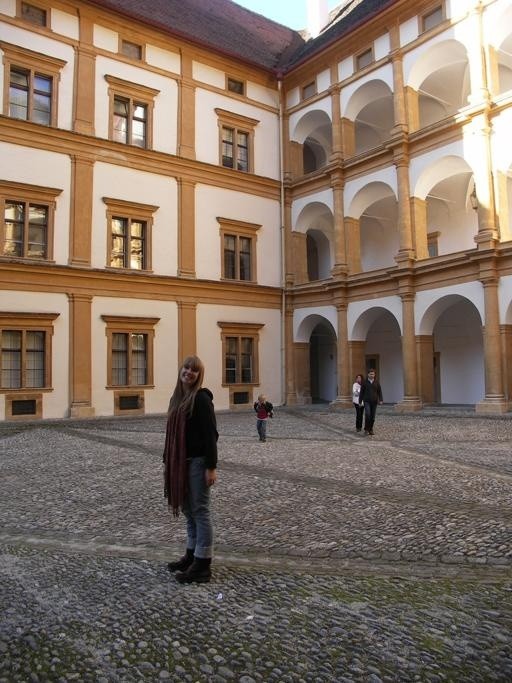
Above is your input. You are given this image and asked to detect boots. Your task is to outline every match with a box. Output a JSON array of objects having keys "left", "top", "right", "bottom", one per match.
[
  {"left": 165, "top": 548, "right": 195, "bottom": 571},
  {"left": 175, "top": 555, "right": 212, "bottom": 584}
]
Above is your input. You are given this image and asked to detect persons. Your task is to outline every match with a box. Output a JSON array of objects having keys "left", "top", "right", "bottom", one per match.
[
  {"left": 160, "top": 353, "right": 220, "bottom": 584},
  {"left": 351, "top": 374, "right": 366, "bottom": 432},
  {"left": 254, "top": 394, "right": 274, "bottom": 442},
  {"left": 359, "top": 369, "right": 383, "bottom": 436}
]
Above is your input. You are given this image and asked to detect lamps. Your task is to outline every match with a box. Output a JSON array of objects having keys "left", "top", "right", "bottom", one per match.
[{"left": 470, "top": 183, "right": 478, "bottom": 212}]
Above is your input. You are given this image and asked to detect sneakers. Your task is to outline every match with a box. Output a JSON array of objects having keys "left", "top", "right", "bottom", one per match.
[{"left": 355, "top": 428, "right": 375, "bottom": 436}]
